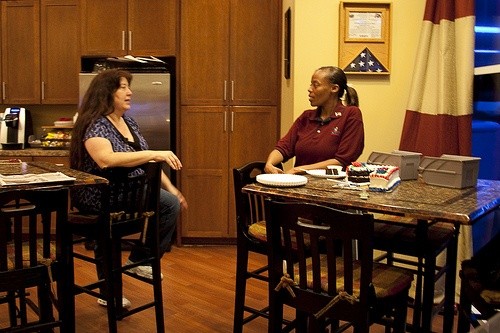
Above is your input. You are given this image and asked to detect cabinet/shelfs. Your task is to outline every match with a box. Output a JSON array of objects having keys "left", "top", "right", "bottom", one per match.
[
  {"left": 182, "top": 106, "right": 277, "bottom": 237},
  {"left": 0, "top": 0, "right": 85, "bottom": 104},
  {"left": 85, "top": 0, "right": 175, "bottom": 56},
  {"left": 180, "top": 0, "right": 278, "bottom": 106}
]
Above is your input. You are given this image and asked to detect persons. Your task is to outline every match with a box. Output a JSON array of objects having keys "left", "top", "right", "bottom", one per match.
[
  {"left": 71, "top": 70, "right": 188, "bottom": 308},
  {"left": 265, "top": 66, "right": 364, "bottom": 175}
]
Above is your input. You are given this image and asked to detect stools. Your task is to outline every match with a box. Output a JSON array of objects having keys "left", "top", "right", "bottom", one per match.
[{"left": 0, "top": 162, "right": 164, "bottom": 333}]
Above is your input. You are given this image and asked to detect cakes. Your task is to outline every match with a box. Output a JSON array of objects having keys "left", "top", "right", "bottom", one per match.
[
  {"left": 346, "top": 162, "right": 401, "bottom": 192},
  {"left": 326, "top": 165, "right": 343, "bottom": 175}
]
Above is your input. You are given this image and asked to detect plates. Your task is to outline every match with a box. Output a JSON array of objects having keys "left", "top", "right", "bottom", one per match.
[
  {"left": 255, "top": 173, "right": 308, "bottom": 187},
  {"left": 306, "top": 169, "right": 346, "bottom": 179}
]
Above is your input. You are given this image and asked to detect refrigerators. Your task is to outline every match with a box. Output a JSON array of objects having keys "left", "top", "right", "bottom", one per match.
[{"left": 78, "top": 73, "right": 172, "bottom": 190}]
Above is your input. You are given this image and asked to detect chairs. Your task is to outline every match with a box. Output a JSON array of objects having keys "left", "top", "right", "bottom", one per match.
[{"left": 233, "top": 162, "right": 500, "bottom": 333}]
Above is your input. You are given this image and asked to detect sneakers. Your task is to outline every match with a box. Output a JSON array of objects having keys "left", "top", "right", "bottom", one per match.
[
  {"left": 97, "top": 295, "right": 132, "bottom": 308},
  {"left": 123, "top": 259, "right": 163, "bottom": 280}
]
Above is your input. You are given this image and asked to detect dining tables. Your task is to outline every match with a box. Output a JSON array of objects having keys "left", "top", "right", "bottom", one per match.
[
  {"left": 0, "top": 162, "right": 108, "bottom": 333},
  {"left": 243, "top": 173, "right": 500, "bottom": 333}
]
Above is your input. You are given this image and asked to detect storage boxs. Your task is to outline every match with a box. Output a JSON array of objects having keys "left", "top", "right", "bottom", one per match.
[
  {"left": 418, "top": 153, "right": 482, "bottom": 188},
  {"left": 366, "top": 149, "right": 423, "bottom": 181}
]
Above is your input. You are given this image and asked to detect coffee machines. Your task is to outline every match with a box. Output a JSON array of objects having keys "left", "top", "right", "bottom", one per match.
[{"left": 0, "top": 107, "right": 32, "bottom": 149}]
